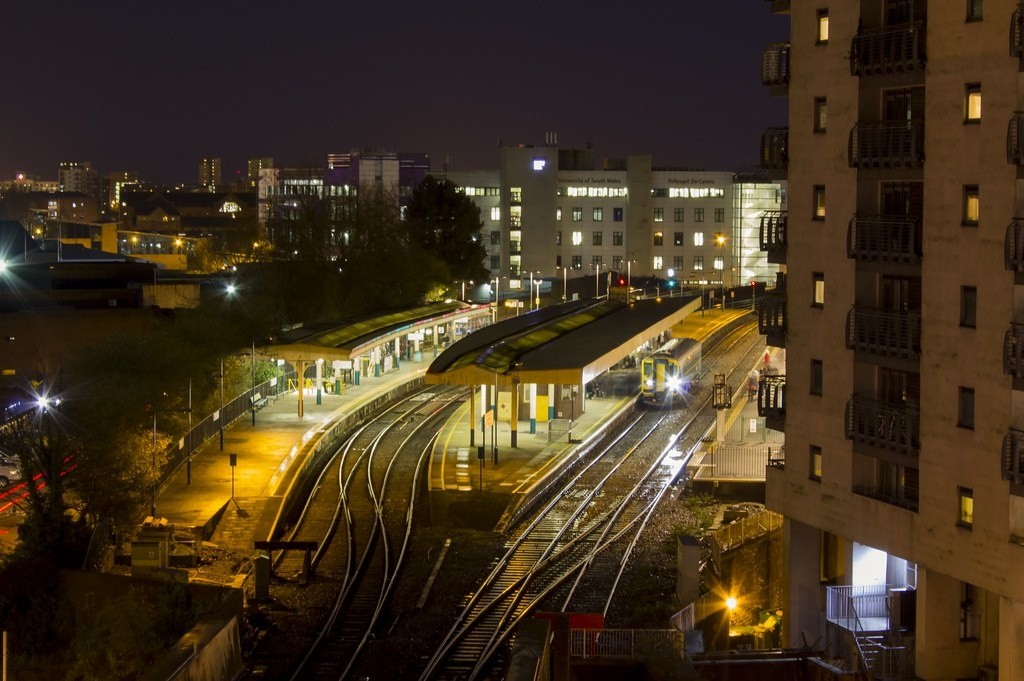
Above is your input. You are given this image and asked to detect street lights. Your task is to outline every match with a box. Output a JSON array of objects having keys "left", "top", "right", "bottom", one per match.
[
  {"left": 522, "top": 270, "right": 540, "bottom": 312},
  {"left": 718, "top": 237, "right": 725, "bottom": 310},
  {"left": 589, "top": 263, "right": 606, "bottom": 299},
  {"left": 488, "top": 274, "right": 507, "bottom": 322},
  {"left": 620, "top": 260, "right": 636, "bottom": 305},
  {"left": 151, "top": 408, "right": 193, "bottom": 518},
  {"left": 218, "top": 350, "right": 251, "bottom": 452},
  {"left": 452, "top": 280, "right": 474, "bottom": 302},
  {"left": 556, "top": 266, "right": 573, "bottom": 302}
]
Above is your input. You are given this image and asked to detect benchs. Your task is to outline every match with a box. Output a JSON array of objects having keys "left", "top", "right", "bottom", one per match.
[{"left": 251, "top": 392, "right": 269, "bottom": 412}]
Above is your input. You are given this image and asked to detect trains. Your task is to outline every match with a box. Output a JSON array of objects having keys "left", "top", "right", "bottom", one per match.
[{"left": 640, "top": 338, "right": 702, "bottom": 411}]
